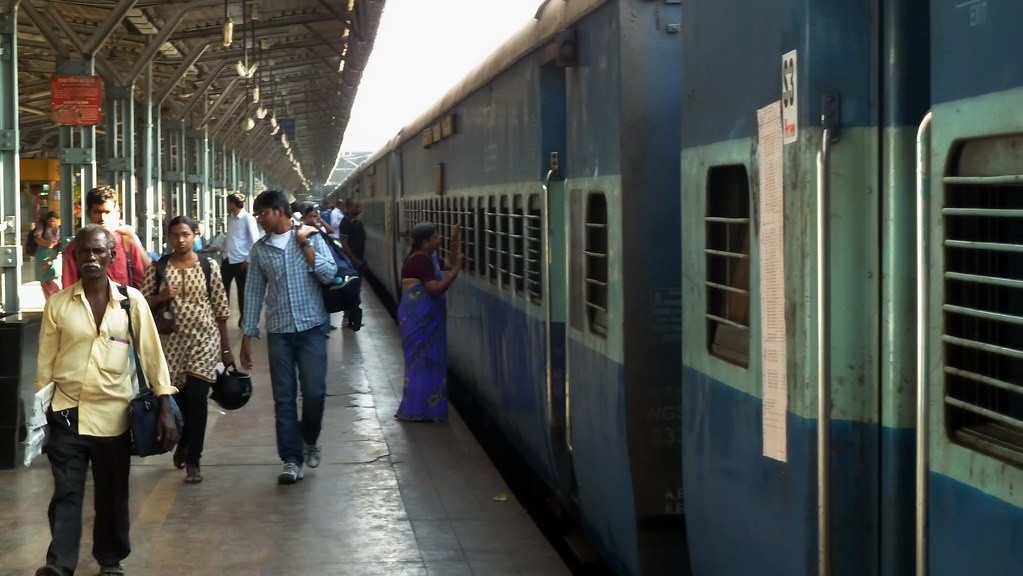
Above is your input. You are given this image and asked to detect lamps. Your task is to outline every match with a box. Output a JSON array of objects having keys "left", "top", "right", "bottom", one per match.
[
  {"left": 241, "top": 117, "right": 255, "bottom": 131},
  {"left": 224, "top": 0, "right": 233, "bottom": 43}
]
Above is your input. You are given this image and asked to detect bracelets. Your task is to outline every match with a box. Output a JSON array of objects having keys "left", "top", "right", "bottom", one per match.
[
  {"left": 300, "top": 239, "right": 311, "bottom": 250},
  {"left": 224, "top": 349, "right": 231, "bottom": 353}
]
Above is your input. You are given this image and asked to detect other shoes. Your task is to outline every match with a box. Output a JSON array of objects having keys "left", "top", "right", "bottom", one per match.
[
  {"left": 99, "top": 561, "right": 124, "bottom": 576},
  {"left": 324, "top": 333, "right": 330, "bottom": 338},
  {"left": 36, "top": 565, "right": 71, "bottom": 576},
  {"left": 173, "top": 439, "right": 188, "bottom": 469},
  {"left": 185, "top": 463, "right": 202, "bottom": 483},
  {"left": 330, "top": 326, "right": 337, "bottom": 330},
  {"left": 342, "top": 316, "right": 365, "bottom": 327}
]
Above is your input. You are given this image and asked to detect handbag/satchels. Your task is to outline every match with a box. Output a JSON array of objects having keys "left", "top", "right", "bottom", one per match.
[
  {"left": 129, "top": 389, "right": 184, "bottom": 457},
  {"left": 150, "top": 260, "right": 176, "bottom": 334},
  {"left": 26, "top": 221, "right": 46, "bottom": 256}
]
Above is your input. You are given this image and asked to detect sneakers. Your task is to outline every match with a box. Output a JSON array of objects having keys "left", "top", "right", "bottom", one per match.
[
  {"left": 297, "top": 422, "right": 322, "bottom": 468},
  {"left": 278, "top": 462, "right": 304, "bottom": 483}
]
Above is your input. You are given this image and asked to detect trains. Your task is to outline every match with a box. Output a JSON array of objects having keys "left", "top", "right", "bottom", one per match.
[{"left": 326, "top": 0, "right": 1023, "bottom": 576}]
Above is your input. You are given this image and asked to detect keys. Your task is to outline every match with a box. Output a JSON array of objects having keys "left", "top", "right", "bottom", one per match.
[{"left": 65, "top": 415, "right": 70, "bottom": 426}]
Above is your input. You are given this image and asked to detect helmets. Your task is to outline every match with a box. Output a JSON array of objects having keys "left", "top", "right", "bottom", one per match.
[{"left": 208, "top": 369, "right": 252, "bottom": 410}]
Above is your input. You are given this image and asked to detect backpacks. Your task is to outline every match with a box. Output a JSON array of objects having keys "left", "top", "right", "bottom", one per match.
[{"left": 295, "top": 222, "right": 360, "bottom": 313}]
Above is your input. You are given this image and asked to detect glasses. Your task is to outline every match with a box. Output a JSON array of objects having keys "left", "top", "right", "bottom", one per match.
[{"left": 253, "top": 208, "right": 273, "bottom": 219}]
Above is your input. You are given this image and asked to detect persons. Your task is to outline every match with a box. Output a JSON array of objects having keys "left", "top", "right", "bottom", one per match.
[
  {"left": 290, "top": 198, "right": 365, "bottom": 336},
  {"left": 115, "top": 225, "right": 160, "bottom": 265},
  {"left": 202, "top": 194, "right": 259, "bottom": 328},
  {"left": 239, "top": 190, "right": 337, "bottom": 482},
  {"left": 62, "top": 186, "right": 144, "bottom": 290},
  {"left": 34, "top": 211, "right": 60, "bottom": 299},
  {"left": 35, "top": 225, "right": 179, "bottom": 576},
  {"left": 392, "top": 223, "right": 467, "bottom": 421},
  {"left": 141, "top": 216, "right": 234, "bottom": 483}
]
